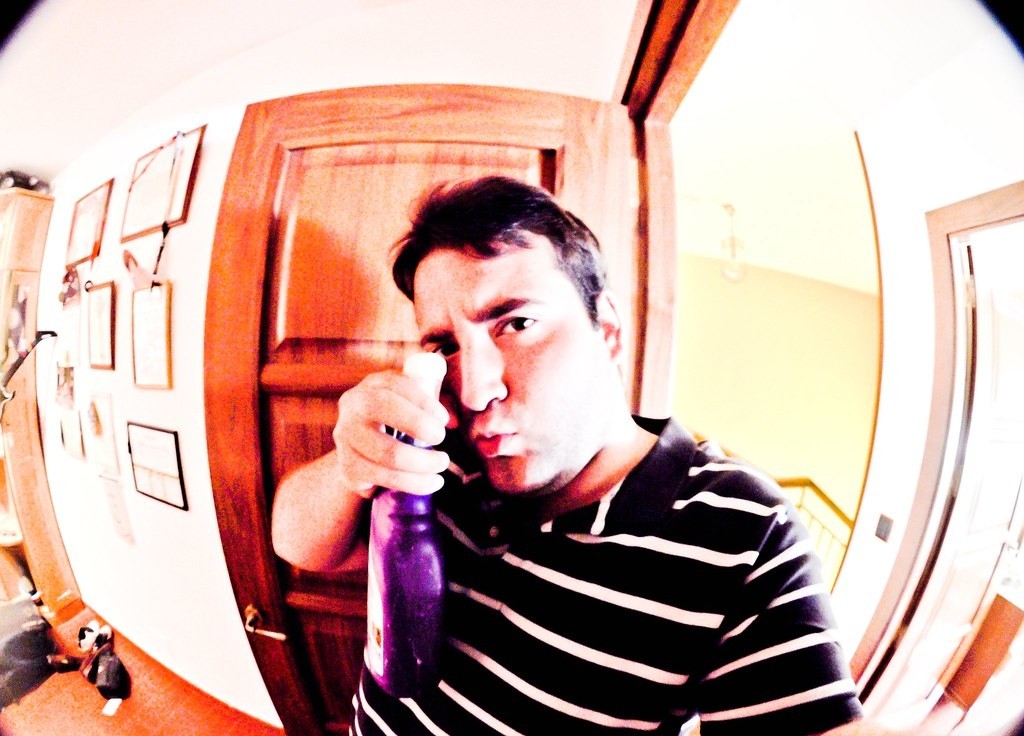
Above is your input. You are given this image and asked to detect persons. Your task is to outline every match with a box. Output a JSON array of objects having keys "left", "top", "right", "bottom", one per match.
[{"left": 272, "top": 176, "right": 963, "bottom": 736}]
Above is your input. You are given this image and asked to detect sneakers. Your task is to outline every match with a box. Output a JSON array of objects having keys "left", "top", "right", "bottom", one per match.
[
  {"left": 92, "top": 625, "right": 112, "bottom": 651},
  {"left": 77, "top": 620, "right": 98, "bottom": 652}
]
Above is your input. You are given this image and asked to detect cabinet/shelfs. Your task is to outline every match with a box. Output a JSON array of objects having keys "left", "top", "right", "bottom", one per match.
[{"left": 0, "top": 187, "right": 87, "bottom": 627}]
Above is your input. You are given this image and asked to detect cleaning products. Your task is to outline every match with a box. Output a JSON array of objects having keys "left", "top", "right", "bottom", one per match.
[{"left": 362, "top": 352, "right": 452, "bottom": 698}]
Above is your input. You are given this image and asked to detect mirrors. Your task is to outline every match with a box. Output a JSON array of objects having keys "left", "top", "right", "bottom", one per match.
[{"left": 0, "top": 1, "right": 1023, "bottom": 736}]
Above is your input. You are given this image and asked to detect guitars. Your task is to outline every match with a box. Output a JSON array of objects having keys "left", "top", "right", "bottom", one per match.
[{"left": 0, "top": 330, "right": 58, "bottom": 421}]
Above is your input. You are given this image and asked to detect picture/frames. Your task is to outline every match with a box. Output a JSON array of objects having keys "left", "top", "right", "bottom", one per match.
[
  {"left": 120, "top": 124, "right": 204, "bottom": 243},
  {"left": 131, "top": 281, "right": 175, "bottom": 389},
  {"left": 128, "top": 421, "right": 188, "bottom": 509},
  {"left": 87, "top": 281, "right": 115, "bottom": 370},
  {"left": 64, "top": 178, "right": 115, "bottom": 273}
]
